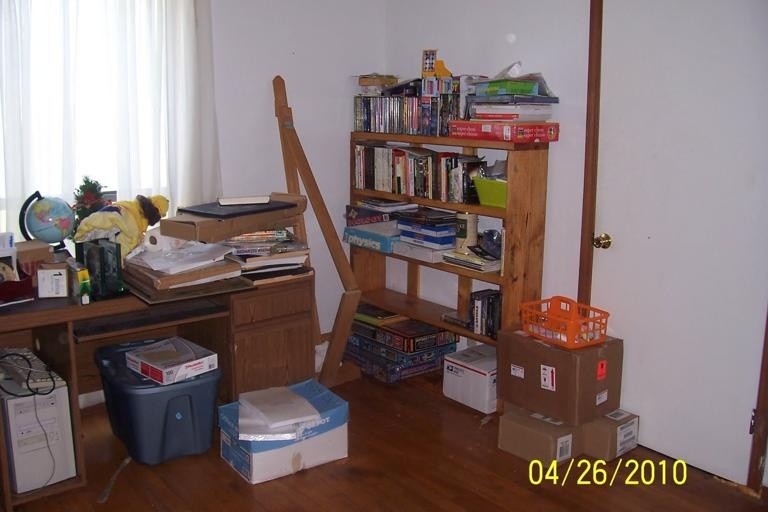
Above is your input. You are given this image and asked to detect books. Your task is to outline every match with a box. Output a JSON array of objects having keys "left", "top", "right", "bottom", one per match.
[
  {"left": 355, "top": 96, "right": 559, "bottom": 137},
  {"left": 359, "top": 75, "right": 398, "bottom": 86},
  {"left": 225, "top": 249, "right": 314, "bottom": 285},
  {"left": 357, "top": 198, "right": 418, "bottom": 212},
  {"left": 354, "top": 145, "right": 487, "bottom": 204},
  {"left": 442, "top": 246, "right": 501, "bottom": 273},
  {"left": 441, "top": 289, "right": 500, "bottom": 339}
]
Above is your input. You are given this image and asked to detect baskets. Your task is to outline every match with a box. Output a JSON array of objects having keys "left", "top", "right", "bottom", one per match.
[{"left": 519, "top": 295, "right": 610, "bottom": 350}]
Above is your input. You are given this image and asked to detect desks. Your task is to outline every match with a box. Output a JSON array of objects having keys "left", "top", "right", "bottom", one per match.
[{"left": 0, "top": 267, "right": 315, "bottom": 512}]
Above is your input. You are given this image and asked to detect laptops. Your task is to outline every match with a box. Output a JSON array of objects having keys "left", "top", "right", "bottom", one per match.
[{"left": 177, "top": 200, "right": 297, "bottom": 219}]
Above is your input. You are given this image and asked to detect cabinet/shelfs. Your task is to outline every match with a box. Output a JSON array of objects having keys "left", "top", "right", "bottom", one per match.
[{"left": 348, "top": 131, "right": 549, "bottom": 416}]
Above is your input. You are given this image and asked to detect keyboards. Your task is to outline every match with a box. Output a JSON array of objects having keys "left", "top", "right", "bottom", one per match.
[{"left": 0, "top": 344, "right": 67, "bottom": 390}]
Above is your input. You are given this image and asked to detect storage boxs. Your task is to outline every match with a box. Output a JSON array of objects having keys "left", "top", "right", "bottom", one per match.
[
  {"left": 96, "top": 336, "right": 221, "bottom": 465},
  {"left": 443, "top": 344, "right": 500, "bottom": 414},
  {"left": 494, "top": 328, "right": 639, "bottom": 468},
  {"left": 219, "top": 378, "right": 347, "bottom": 485},
  {"left": 14, "top": 240, "right": 54, "bottom": 288}
]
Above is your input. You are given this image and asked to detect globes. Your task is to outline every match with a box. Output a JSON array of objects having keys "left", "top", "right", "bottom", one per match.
[{"left": 19, "top": 191, "right": 76, "bottom": 263}]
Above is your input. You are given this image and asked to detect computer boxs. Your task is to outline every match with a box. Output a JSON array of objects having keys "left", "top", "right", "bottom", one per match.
[{"left": 0, "top": 373, "right": 77, "bottom": 494}]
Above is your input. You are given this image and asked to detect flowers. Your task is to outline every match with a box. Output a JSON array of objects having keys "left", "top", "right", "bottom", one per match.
[{"left": 67, "top": 175, "right": 113, "bottom": 240}]
